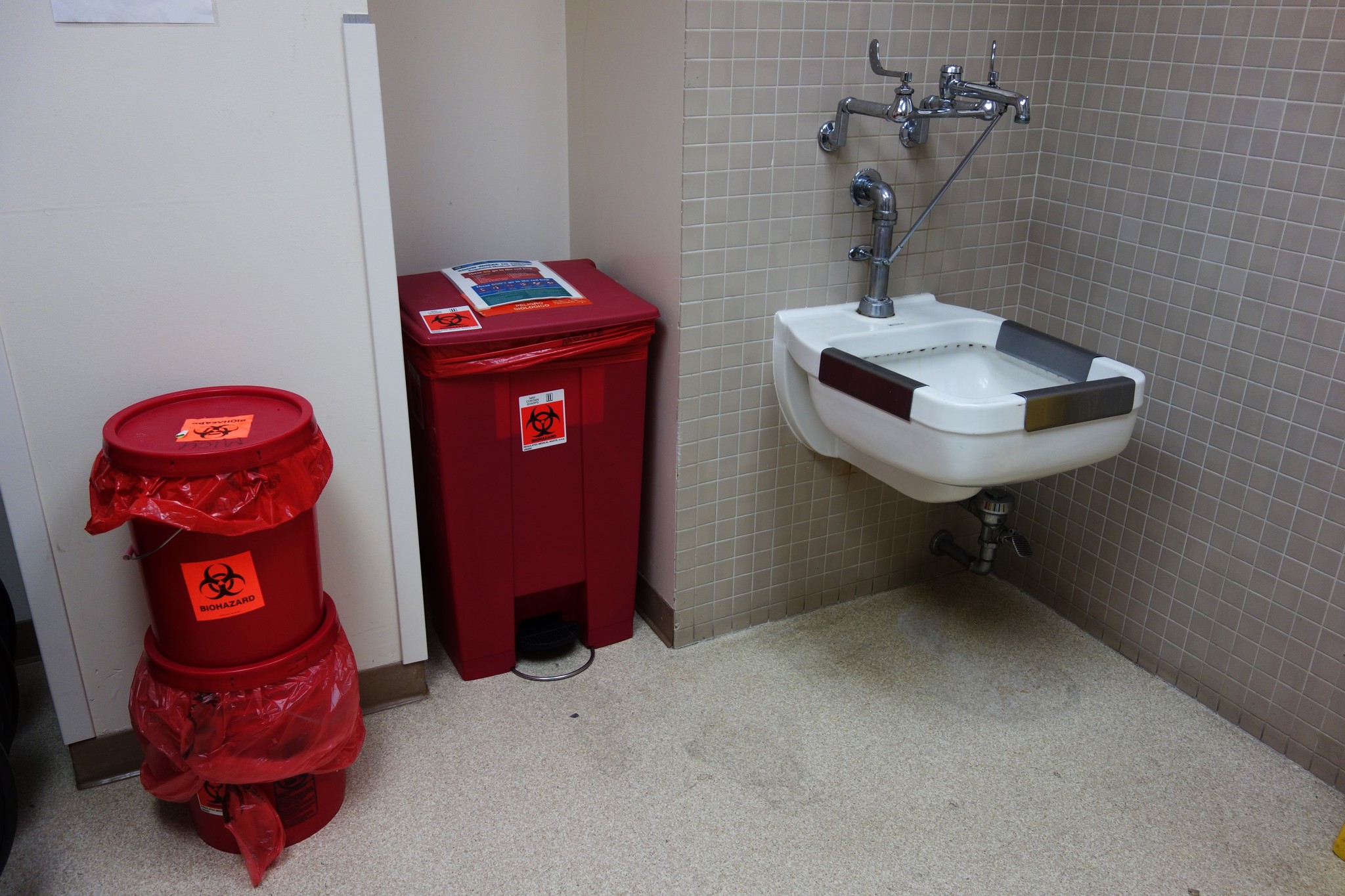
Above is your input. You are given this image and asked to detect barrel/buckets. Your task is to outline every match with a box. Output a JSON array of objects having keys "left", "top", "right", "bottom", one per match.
[
  {"left": 106, "top": 387, "right": 325, "bottom": 669},
  {"left": 141, "top": 597, "right": 345, "bottom": 856}
]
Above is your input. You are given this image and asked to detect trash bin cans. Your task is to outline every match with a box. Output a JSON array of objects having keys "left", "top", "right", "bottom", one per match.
[
  {"left": 128, "top": 591, "right": 364, "bottom": 890},
  {"left": 82, "top": 385, "right": 335, "bottom": 668},
  {"left": 398, "top": 259, "right": 661, "bottom": 682}
]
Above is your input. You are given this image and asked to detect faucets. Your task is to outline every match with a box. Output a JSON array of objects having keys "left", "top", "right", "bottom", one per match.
[{"left": 939, "top": 64, "right": 1033, "bottom": 125}]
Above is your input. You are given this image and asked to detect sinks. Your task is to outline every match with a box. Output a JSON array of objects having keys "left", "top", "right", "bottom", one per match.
[{"left": 774, "top": 292, "right": 1147, "bottom": 505}]
